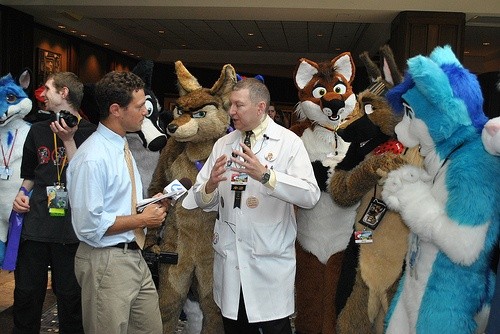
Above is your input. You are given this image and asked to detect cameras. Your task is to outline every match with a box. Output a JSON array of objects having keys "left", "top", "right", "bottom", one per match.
[{"left": 57, "top": 110, "right": 78, "bottom": 128}]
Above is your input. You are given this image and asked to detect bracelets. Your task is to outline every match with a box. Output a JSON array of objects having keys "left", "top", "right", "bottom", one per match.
[{"left": 19, "top": 187, "right": 29, "bottom": 196}]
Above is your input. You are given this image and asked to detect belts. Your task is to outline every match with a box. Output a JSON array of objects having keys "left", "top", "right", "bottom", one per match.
[{"left": 110, "top": 241, "right": 140, "bottom": 250}]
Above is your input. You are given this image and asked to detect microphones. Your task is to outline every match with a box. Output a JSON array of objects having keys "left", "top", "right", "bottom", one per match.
[{"left": 163, "top": 178, "right": 193, "bottom": 206}]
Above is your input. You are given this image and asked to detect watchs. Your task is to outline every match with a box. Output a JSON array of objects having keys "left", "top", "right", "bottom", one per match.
[{"left": 261, "top": 167, "right": 270, "bottom": 183}]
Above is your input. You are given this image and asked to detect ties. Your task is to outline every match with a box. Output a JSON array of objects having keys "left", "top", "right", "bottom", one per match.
[
  {"left": 242, "top": 130, "right": 253, "bottom": 161},
  {"left": 124, "top": 140, "right": 147, "bottom": 251}
]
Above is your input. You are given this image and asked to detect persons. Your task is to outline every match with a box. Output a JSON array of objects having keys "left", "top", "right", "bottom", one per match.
[
  {"left": 268, "top": 102, "right": 276, "bottom": 120},
  {"left": 194, "top": 78, "right": 321, "bottom": 334},
  {"left": 12, "top": 72, "right": 97, "bottom": 334},
  {"left": 65, "top": 69, "right": 172, "bottom": 334}
]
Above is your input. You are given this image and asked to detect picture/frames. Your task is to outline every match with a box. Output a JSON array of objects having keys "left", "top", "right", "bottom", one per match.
[
  {"left": 280, "top": 110, "right": 293, "bottom": 128},
  {"left": 161, "top": 95, "right": 179, "bottom": 112},
  {"left": 36, "top": 48, "right": 62, "bottom": 89}
]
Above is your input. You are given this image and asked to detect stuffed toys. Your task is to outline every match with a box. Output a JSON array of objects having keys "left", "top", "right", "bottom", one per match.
[
  {"left": 281, "top": 51, "right": 358, "bottom": 334},
  {"left": 142, "top": 60, "right": 237, "bottom": 334},
  {"left": 382, "top": 45, "right": 500, "bottom": 334},
  {"left": 482, "top": 116, "right": 500, "bottom": 157},
  {"left": 0, "top": 70, "right": 33, "bottom": 319},
  {"left": 82, "top": 55, "right": 168, "bottom": 291},
  {"left": 333, "top": 45, "right": 426, "bottom": 334}
]
